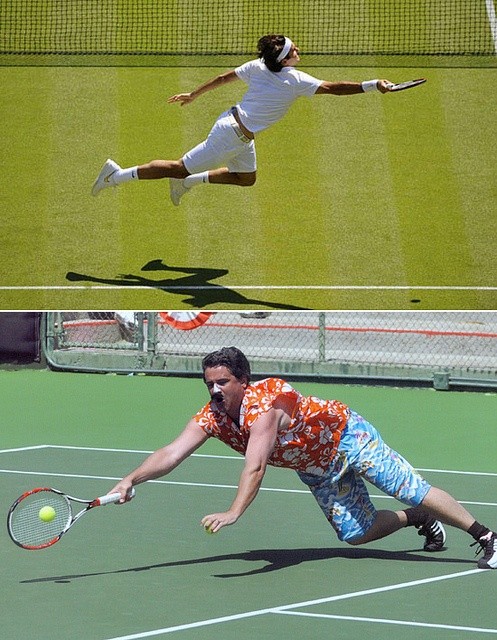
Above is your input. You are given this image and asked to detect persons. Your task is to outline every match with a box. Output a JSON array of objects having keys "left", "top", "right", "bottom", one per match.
[
  {"left": 90, "top": 35, "right": 395, "bottom": 208},
  {"left": 106, "top": 346, "right": 497, "bottom": 569}
]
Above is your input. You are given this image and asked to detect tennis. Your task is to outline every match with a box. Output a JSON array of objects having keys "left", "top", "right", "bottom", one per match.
[
  {"left": 40, "top": 506, "right": 55, "bottom": 521},
  {"left": 204, "top": 522, "right": 219, "bottom": 534}
]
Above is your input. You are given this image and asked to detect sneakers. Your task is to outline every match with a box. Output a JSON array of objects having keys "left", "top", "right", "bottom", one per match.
[
  {"left": 169, "top": 176, "right": 191, "bottom": 206},
  {"left": 91, "top": 159, "right": 121, "bottom": 197},
  {"left": 418, "top": 517, "right": 446, "bottom": 552},
  {"left": 469, "top": 531, "right": 497, "bottom": 569}
]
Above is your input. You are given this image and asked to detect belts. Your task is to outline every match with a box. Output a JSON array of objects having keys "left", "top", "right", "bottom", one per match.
[{"left": 227, "top": 111, "right": 252, "bottom": 143}]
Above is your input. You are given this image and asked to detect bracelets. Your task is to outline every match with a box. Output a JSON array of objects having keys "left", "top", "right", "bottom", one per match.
[{"left": 362, "top": 79, "right": 379, "bottom": 93}]
